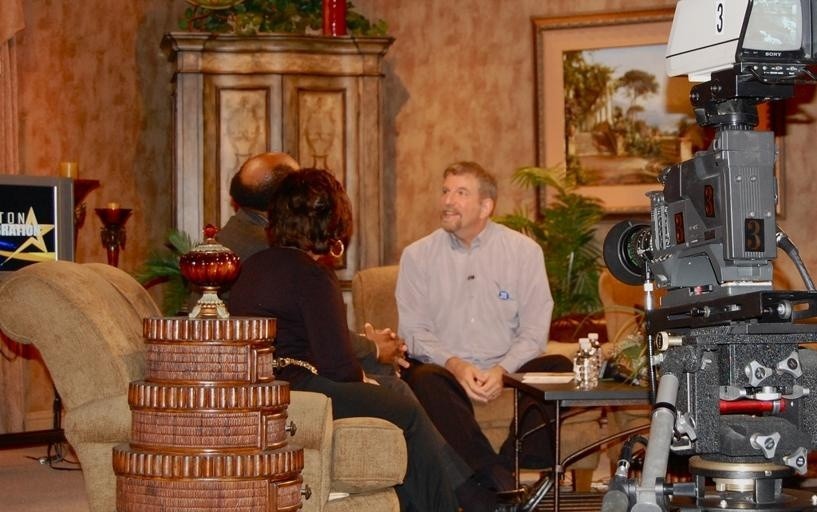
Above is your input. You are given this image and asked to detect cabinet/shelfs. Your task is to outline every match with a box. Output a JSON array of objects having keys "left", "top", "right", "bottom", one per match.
[
  {"left": 160, "top": 31, "right": 396, "bottom": 330},
  {"left": 1, "top": 273, "right": 67, "bottom": 434}
]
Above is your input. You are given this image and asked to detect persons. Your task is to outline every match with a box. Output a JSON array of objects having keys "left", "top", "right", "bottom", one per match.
[
  {"left": 219, "top": 168, "right": 470, "bottom": 511},
  {"left": 213, "top": 149, "right": 411, "bottom": 377},
  {"left": 393, "top": 162, "right": 576, "bottom": 511}
]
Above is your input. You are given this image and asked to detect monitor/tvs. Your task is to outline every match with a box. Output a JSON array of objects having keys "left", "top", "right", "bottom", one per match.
[{"left": 0, "top": 174, "right": 75, "bottom": 282}]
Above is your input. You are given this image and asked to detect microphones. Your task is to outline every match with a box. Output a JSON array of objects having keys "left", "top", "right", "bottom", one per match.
[{"left": 610, "top": 364, "right": 641, "bottom": 386}]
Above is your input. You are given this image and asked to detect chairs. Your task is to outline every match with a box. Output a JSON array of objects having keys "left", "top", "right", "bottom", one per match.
[
  {"left": 352, "top": 265, "right": 602, "bottom": 494},
  {"left": 598, "top": 269, "right": 817, "bottom": 478},
  {"left": 1, "top": 260, "right": 408, "bottom": 512}
]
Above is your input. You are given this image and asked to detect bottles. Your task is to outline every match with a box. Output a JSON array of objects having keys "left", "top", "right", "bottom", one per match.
[{"left": 574, "top": 333, "right": 607, "bottom": 391}]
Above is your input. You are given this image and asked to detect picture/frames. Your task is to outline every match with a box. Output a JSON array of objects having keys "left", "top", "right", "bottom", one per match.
[{"left": 532, "top": 10, "right": 786, "bottom": 221}]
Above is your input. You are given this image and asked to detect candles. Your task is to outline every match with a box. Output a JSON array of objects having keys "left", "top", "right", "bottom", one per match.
[
  {"left": 107, "top": 202, "right": 121, "bottom": 209},
  {"left": 62, "top": 162, "right": 78, "bottom": 179}
]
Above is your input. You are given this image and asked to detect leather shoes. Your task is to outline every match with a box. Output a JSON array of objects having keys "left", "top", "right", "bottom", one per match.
[{"left": 494, "top": 475, "right": 554, "bottom": 512}]
